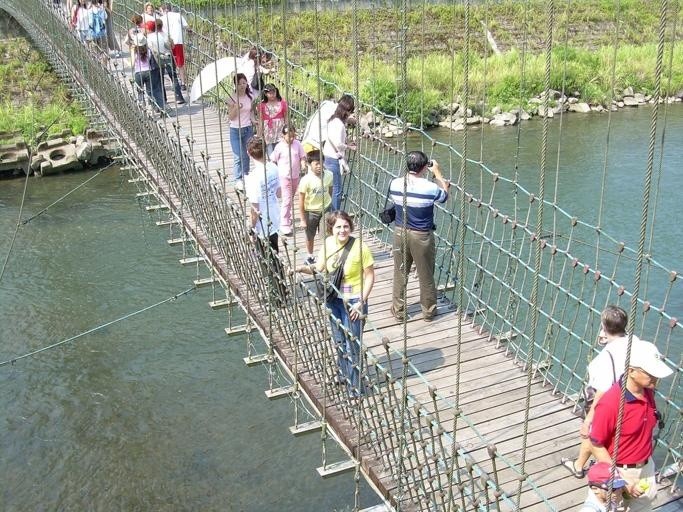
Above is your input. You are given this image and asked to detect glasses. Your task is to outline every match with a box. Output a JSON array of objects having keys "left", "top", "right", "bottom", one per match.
[{"left": 654, "top": 410, "right": 664, "bottom": 430}]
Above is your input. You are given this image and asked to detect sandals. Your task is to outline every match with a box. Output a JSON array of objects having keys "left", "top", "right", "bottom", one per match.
[{"left": 561, "top": 457, "right": 584, "bottom": 479}]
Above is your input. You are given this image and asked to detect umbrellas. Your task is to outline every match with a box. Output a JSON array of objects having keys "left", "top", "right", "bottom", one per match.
[{"left": 191, "top": 57, "right": 246, "bottom": 104}]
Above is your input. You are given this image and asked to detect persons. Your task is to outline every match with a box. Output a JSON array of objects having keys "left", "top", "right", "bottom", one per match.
[
  {"left": 225, "top": 73, "right": 256, "bottom": 191},
  {"left": 53, "top": 2, "right": 190, "bottom": 119},
  {"left": 287, "top": 210, "right": 376, "bottom": 397},
  {"left": 559, "top": 304, "right": 642, "bottom": 481},
  {"left": 296, "top": 150, "right": 335, "bottom": 265},
  {"left": 322, "top": 96, "right": 358, "bottom": 238},
  {"left": 256, "top": 83, "right": 289, "bottom": 164},
  {"left": 270, "top": 123, "right": 306, "bottom": 237},
  {"left": 245, "top": 135, "right": 288, "bottom": 309},
  {"left": 588, "top": 341, "right": 675, "bottom": 511},
  {"left": 244, "top": 46, "right": 278, "bottom": 92},
  {"left": 303, "top": 92, "right": 352, "bottom": 156},
  {"left": 388, "top": 151, "right": 450, "bottom": 324},
  {"left": 580, "top": 462, "right": 631, "bottom": 512}
]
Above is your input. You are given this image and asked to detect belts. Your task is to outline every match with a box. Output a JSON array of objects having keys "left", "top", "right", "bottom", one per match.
[{"left": 616, "top": 460, "right": 648, "bottom": 468}]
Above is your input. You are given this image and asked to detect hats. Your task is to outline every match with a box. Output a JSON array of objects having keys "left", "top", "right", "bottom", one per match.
[
  {"left": 586, "top": 462, "right": 628, "bottom": 488},
  {"left": 629, "top": 340, "right": 673, "bottom": 379},
  {"left": 140, "top": 20, "right": 155, "bottom": 32}
]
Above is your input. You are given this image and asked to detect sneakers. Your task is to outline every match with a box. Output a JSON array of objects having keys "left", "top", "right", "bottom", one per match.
[
  {"left": 279, "top": 225, "right": 294, "bottom": 236},
  {"left": 236, "top": 180, "right": 244, "bottom": 190},
  {"left": 391, "top": 306, "right": 404, "bottom": 322},
  {"left": 304, "top": 256, "right": 316, "bottom": 265},
  {"left": 176, "top": 95, "right": 186, "bottom": 104},
  {"left": 424, "top": 315, "right": 432, "bottom": 322},
  {"left": 180, "top": 85, "right": 187, "bottom": 91}
]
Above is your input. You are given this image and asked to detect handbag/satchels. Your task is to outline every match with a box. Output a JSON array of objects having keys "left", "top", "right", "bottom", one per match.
[
  {"left": 338, "top": 158, "right": 350, "bottom": 176},
  {"left": 317, "top": 267, "right": 342, "bottom": 304},
  {"left": 251, "top": 71, "right": 264, "bottom": 90},
  {"left": 379, "top": 206, "right": 395, "bottom": 223}
]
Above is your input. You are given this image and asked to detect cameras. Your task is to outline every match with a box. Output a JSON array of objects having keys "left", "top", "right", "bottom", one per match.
[{"left": 428, "top": 161, "right": 433, "bottom": 167}]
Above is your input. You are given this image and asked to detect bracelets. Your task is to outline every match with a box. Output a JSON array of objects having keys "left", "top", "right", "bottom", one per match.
[{"left": 337, "top": 157, "right": 344, "bottom": 160}]
